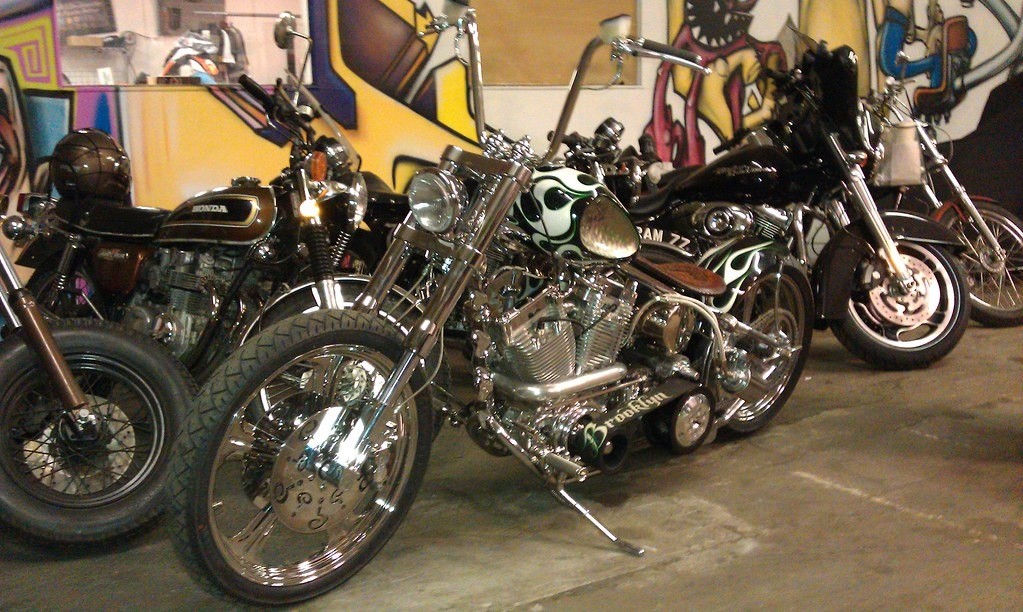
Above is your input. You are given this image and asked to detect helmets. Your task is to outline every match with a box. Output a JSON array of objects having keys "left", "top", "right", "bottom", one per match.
[{"left": 49, "top": 128, "right": 130, "bottom": 201}]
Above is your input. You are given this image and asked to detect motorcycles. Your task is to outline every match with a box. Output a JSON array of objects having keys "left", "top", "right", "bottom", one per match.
[{"left": 1, "top": 8, "right": 1023, "bottom": 611}]
